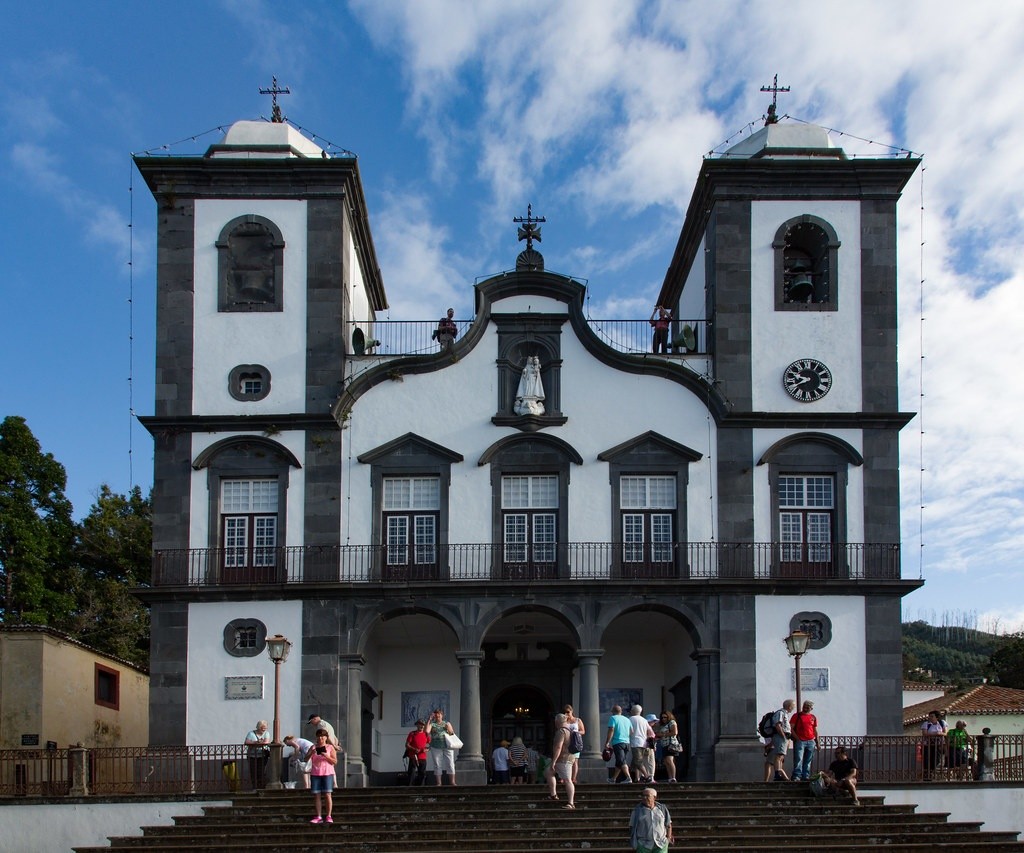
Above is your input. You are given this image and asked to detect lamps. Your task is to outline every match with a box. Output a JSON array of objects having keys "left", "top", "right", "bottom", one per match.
[{"left": 515, "top": 704, "right": 530, "bottom": 717}]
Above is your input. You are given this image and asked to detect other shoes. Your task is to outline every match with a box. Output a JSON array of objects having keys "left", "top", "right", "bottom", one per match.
[
  {"left": 668, "top": 778, "right": 676, "bottom": 782},
  {"left": 607, "top": 777, "right": 615, "bottom": 784},
  {"left": 774, "top": 776, "right": 785, "bottom": 781},
  {"left": 326, "top": 817, "right": 333, "bottom": 823},
  {"left": 620, "top": 777, "right": 633, "bottom": 784},
  {"left": 310, "top": 817, "right": 325, "bottom": 824},
  {"left": 851, "top": 800, "right": 860, "bottom": 804}
]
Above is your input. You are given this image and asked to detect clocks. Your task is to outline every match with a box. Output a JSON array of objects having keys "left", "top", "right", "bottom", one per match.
[{"left": 783, "top": 358, "right": 833, "bottom": 402}]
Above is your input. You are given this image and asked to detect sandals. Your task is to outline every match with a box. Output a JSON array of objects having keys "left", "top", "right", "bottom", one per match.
[
  {"left": 562, "top": 802, "right": 575, "bottom": 809},
  {"left": 543, "top": 794, "right": 558, "bottom": 800}
]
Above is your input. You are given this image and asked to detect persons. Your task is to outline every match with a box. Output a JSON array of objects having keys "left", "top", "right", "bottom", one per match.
[
  {"left": 492, "top": 737, "right": 539, "bottom": 785},
  {"left": 762, "top": 699, "right": 795, "bottom": 781},
  {"left": 790, "top": 700, "right": 822, "bottom": 781},
  {"left": 304, "top": 729, "right": 338, "bottom": 822},
  {"left": 543, "top": 714, "right": 575, "bottom": 809},
  {"left": 628, "top": 704, "right": 652, "bottom": 782},
  {"left": 656, "top": 710, "right": 683, "bottom": 782},
  {"left": 920, "top": 711, "right": 969, "bottom": 781},
  {"left": 558, "top": 704, "right": 585, "bottom": 784},
  {"left": 438, "top": 307, "right": 458, "bottom": 349},
  {"left": 649, "top": 306, "right": 672, "bottom": 353},
  {"left": 822, "top": 746, "right": 860, "bottom": 805},
  {"left": 639, "top": 714, "right": 660, "bottom": 783},
  {"left": 604, "top": 705, "right": 634, "bottom": 783},
  {"left": 283, "top": 736, "right": 314, "bottom": 788},
  {"left": 404, "top": 718, "right": 431, "bottom": 787},
  {"left": 629, "top": 788, "right": 674, "bottom": 853},
  {"left": 305, "top": 713, "right": 341, "bottom": 788},
  {"left": 245, "top": 720, "right": 270, "bottom": 789},
  {"left": 517, "top": 357, "right": 546, "bottom": 402},
  {"left": 424, "top": 710, "right": 456, "bottom": 786}
]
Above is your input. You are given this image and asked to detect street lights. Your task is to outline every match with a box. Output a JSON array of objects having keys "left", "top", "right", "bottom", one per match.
[
  {"left": 263, "top": 632, "right": 293, "bottom": 791},
  {"left": 782, "top": 628, "right": 810, "bottom": 713}
]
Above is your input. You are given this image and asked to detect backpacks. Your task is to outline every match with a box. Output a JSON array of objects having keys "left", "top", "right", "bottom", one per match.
[
  {"left": 561, "top": 726, "right": 583, "bottom": 754},
  {"left": 809, "top": 770, "right": 828, "bottom": 796},
  {"left": 759, "top": 711, "right": 785, "bottom": 737}
]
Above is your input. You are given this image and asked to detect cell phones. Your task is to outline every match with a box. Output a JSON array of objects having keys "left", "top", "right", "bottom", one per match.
[{"left": 316, "top": 746, "right": 327, "bottom": 755}]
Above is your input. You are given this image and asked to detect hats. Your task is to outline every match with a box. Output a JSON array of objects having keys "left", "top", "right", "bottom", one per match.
[
  {"left": 501, "top": 740, "right": 510, "bottom": 746},
  {"left": 307, "top": 713, "right": 318, "bottom": 725},
  {"left": 414, "top": 719, "right": 426, "bottom": 725},
  {"left": 645, "top": 713, "right": 660, "bottom": 724}
]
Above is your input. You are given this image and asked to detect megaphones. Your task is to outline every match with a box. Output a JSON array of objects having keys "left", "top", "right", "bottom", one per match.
[
  {"left": 351, "top": 327, "right": 382, "bottom": 357},
  {"left": 666, "top": 324, "right": 695, "bottom": 351}
]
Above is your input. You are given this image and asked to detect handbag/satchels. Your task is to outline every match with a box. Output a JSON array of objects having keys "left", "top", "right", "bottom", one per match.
[
  {"left": 305, "top": 745, "right": 316, "bottom": 773},
  {"left": 668, "top": 743, "right": 684, "bottom": 752},
  {"left": 444, "top": 729, "right": 463, "bottom": 750},
  {"left": 403, "top": 748, "right": 412, "bottom": 759},
  {"left": 296, "top": 759, "right": 309, "bottom": 775}
]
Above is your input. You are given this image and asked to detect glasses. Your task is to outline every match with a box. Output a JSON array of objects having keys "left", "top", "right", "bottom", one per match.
[
  {"left": 563, "top": 709, "right": 571, "bottom": 714},
  {"left": 662, "top": 716, "right": 668, "bottom": 719},
  {"left": 835, "top": 752, "right": 841, "bottom": 754}
]
